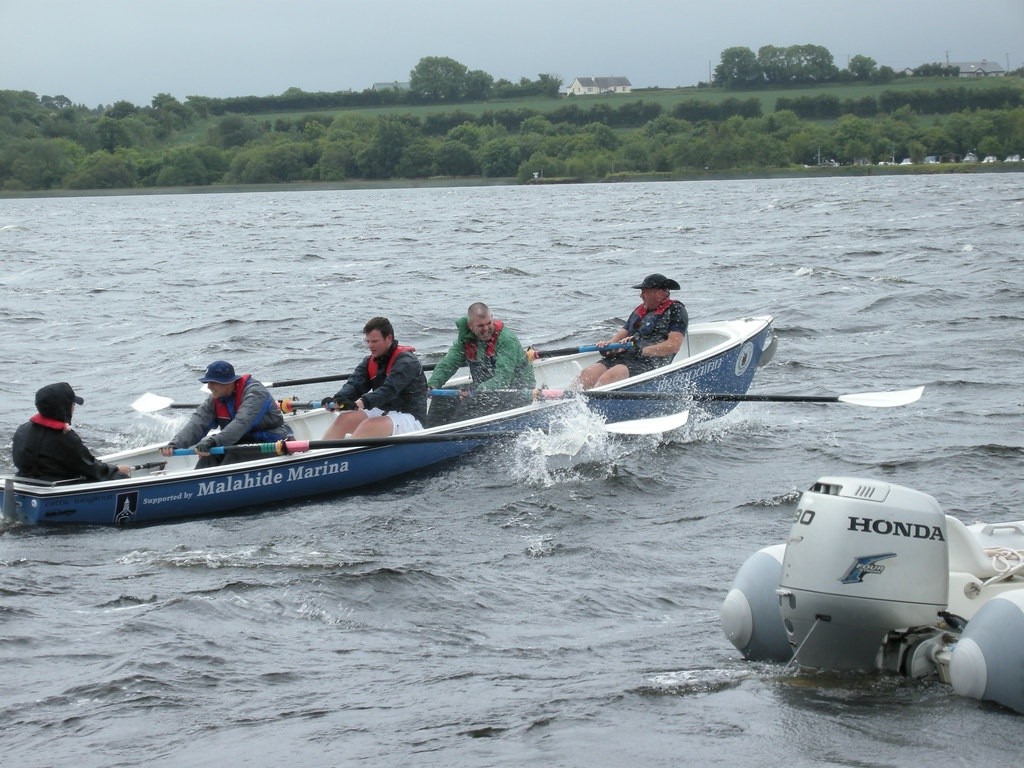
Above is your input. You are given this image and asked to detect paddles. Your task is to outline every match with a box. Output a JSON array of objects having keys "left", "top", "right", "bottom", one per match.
[
  {"left": 158, "top": 407, "right": 691, "bottom": 458},
  {"left": 131, "top": 391, "right": 343, "bottom": 414},
  {"left": 427, "top": 385, "right": 925, "bottom": 408},
  {"left": 197, "top": 334, "right": 636, "bottom": 396}
]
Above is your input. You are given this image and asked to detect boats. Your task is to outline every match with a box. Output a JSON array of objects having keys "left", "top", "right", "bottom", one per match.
[
  {"left": 0, "top": 312, "right": 776, "bottom": 531},
  {"left": 722, "top": 472, "right": 1024, "bottom": 720}
]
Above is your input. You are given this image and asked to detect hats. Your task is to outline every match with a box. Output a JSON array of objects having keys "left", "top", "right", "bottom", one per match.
[
  {"left": 630, "top": 274, "right": 681, "bottom": 290},
  {"left": 56, "top": 382, "right": 85, "bottom": 405},
  {"left": 198, "top": 361, "right": 243, "bottom": 384}
]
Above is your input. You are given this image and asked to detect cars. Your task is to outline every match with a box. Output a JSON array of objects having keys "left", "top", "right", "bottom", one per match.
[
  {"left": 964, "top": 155, "right": 978, "bottom": 163},
  {"left": 899, "top": 158, "right": 912, "bottom": 165},
  {"left": 1004, "top": 155, "right": 1021, "bottom": 162},
  {"left": 924, "top": 155, "right": 940, "bottom": 163},
  {"left": 982, "top": 156, "right": 998, "bottom": 163}
]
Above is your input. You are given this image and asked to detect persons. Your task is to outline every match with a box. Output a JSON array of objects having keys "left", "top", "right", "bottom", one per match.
[
  {"left": 323, "top": 317, "right": 428, "bottom": 440},
  {"left": 426, "top": 303, "right": 535, "bottom": 428},
  {"left": 13, "top": 382, "right": 130, "bottom": 487},
  {"left": 159, "top": 361, "right": 296, "bottom": 470},
  {"left": 579, "top": 274, "right": 689, "bottom": 390}
]
